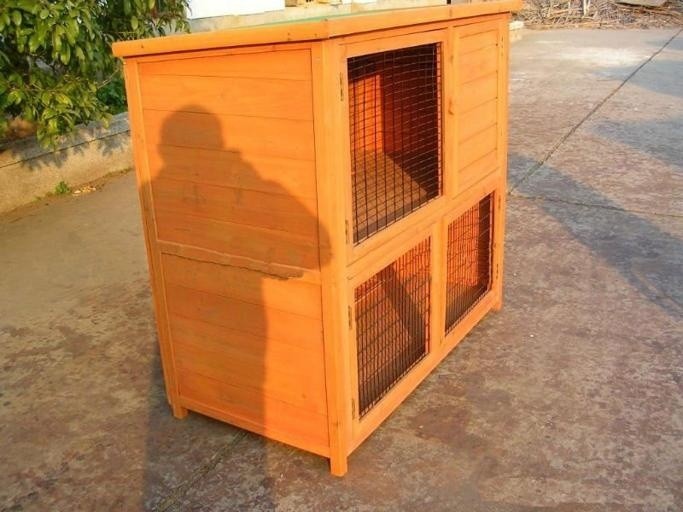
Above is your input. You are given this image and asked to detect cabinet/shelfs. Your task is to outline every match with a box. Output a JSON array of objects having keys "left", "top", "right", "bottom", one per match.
[{"left": 112, "top": 1, "right": 524, "bottom": 477}]
